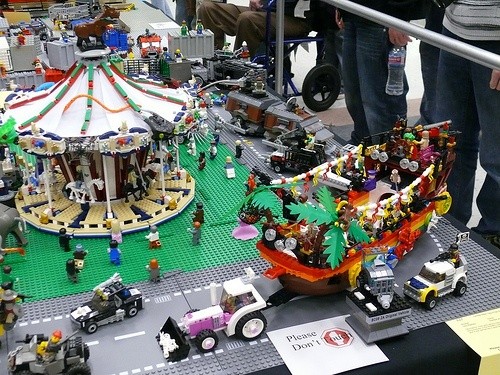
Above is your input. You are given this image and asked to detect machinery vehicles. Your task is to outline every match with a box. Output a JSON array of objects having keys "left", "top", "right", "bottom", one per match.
[{"left": 155, "top": 275, "right": 270, "bottom": 363}]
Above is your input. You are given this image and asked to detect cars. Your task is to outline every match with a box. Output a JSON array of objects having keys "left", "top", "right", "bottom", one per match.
[
  {"left": 6, "top": 333, "right": 92, "bottom": 375},
  {"left": 403, "top": 254, "right": 470, "bottom": 310},
  {"left": 69, "top": 281, "right": 143, "bottom": 334}
]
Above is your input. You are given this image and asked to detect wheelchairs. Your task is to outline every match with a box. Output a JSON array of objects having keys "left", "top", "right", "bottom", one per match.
[{"left": 251, "top": 0, "right": 342, "bottom": 111}]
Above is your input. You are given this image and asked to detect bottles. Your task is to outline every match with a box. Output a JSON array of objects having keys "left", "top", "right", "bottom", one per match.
[{"left": 386, "top": 45, "right": 406, "bottom": 96}]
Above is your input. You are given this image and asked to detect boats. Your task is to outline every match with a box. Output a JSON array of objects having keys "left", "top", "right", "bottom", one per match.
[{"left": 230, "top": 116, "right": 458, "bottom": 296}]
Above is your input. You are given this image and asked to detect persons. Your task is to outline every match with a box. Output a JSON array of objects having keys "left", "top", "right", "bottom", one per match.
[
  {"left": 180, "top": 19, "right": 189, "bottom": 39},
  {"left": 336, "top": 0, "right": 435, "bottom": 154},
  {"left": 60, "top": 26, "right": 69, "bottom": 42},
  {"left": 195, "top": 18, "right": 204, "bottom": 34},
  {"left": 418, "top": 0, "right": 500, "bottom": 246},
  {"left": 0, "top": 41, "right": 459, "bottom": 354},
  {"left": 16, "top": 32, "right": 25, "bottom": 45},
  {"left": 195, "top": 0, "right": 316, "bottom": 62},
  {"left": 149, "top": 0, "right": 226, "bottom": 30}
]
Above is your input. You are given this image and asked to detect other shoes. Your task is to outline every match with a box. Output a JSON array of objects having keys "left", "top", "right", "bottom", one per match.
[
  {"left": 348, "top": 138, "right": 360, "bottom": 146},
  {"left": 336, "top": 87, "right": 345, "bottom": 100},
  {"left": 478, "top": 234, "right": 500, "bottom": 249}
]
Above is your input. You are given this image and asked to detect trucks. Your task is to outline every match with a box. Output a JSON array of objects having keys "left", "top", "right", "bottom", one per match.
[{"left": 270, "top": 143, "right": 327, "bottom": 173}]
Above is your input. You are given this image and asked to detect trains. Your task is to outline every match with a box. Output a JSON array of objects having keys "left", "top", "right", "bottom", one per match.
[{"left": 225, "top": 85, "right": 335, "bottom": 151}]
